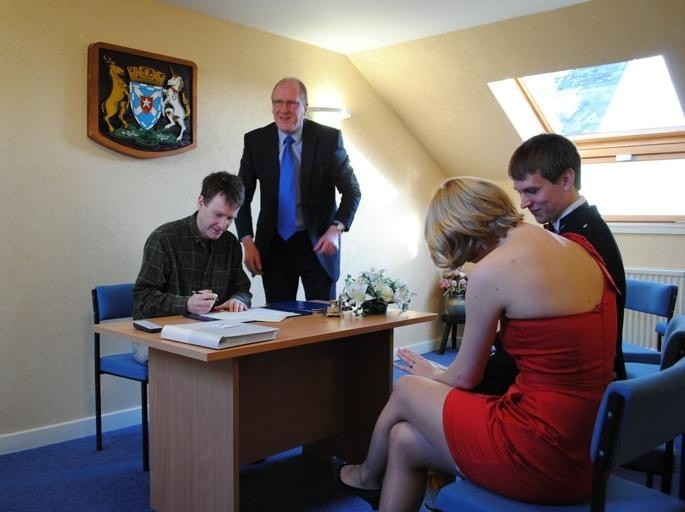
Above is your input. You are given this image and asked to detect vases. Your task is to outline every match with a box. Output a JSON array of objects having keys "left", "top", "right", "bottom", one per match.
[
  {"left": 363, "top": 300, "right": 385, "bottom": 314},
  {"left": 446, "top": 295, "right": 466, "bottom": 314}
]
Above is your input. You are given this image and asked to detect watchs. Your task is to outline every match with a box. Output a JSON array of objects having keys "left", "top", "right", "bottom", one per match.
[{"left": 331, "top": 222, "right": 344, "bottom": 233}]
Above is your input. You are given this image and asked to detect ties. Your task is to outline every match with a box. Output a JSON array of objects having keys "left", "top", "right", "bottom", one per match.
[{"left": 277, "top": 136, "right": 297, "bottom": 240}]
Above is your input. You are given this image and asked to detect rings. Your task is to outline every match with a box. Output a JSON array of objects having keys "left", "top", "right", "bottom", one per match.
[{"left": 410, "top": 362, "right": 414, "bottom": 369}]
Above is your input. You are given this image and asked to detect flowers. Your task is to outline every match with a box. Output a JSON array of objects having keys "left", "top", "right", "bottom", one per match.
[
  {"left": 438, "top": 265, "right": 469, "bottom": 293},
  {"left": 337, "top": 269, "right": 407, "bottom": 306}
]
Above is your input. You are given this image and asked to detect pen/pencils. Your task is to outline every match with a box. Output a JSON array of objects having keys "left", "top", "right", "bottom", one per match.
[{"left": 190, "top": 290, "right": 219, "bottom": 302}]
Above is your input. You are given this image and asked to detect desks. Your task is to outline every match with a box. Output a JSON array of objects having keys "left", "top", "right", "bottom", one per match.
[{"left": 94, "top": 300, "right": 440, "bottom": 512}]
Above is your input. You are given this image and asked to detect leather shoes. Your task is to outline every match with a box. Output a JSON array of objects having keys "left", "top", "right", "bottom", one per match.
[{"left": 331, "top": 456, "right": 380, "bottom": 502}]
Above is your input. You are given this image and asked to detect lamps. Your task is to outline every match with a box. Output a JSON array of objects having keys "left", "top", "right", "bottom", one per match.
[{"left": 307, "top": 84, "right": 347, "bottom": 126}]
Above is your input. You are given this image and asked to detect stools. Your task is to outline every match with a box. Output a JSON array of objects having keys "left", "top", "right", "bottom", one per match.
[{"left": 437, "top": 316, "right": 470, "bottom": 355}]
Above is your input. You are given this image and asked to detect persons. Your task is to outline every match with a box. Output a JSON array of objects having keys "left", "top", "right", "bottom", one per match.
[
  {"left": 235, "top": 78, "right": 360, "bottom": 303},
  {"left": 328, "top": 177, "right": 622, "bottom": 512},
  {"left": 472, "top": 135, "right": 627, "bottom": 396},
  {"left": 132, "top": 172, "right": 252, "bottom": 319}
]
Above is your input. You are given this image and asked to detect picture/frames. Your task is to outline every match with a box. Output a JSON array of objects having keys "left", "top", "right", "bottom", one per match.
[{"left": 86, "top": 42, "right": 197, "bottom": 160}]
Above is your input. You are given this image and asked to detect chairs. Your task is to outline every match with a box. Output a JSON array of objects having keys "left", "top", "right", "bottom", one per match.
[
  {"left": 625, "top": 315, "right": 685, "bottom": 492},
  {"left": 431, "top": 361, "right": 685, "bottom": 512},
  {"left": 92, "top": 284, "right": 147, "bottom": 474},
  {"left": 619, "top": 278, "right": 677, "bottom": 361}
]
